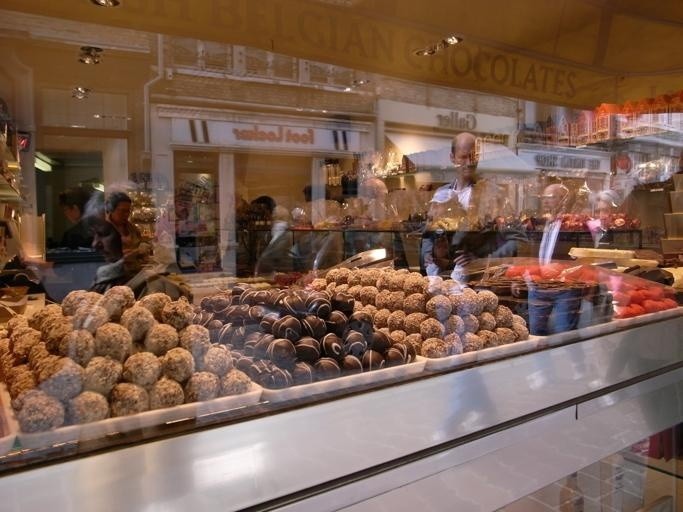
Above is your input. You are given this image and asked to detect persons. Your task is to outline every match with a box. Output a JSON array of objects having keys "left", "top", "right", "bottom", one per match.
[
  {"left": 512, "top": 184, "right": 595, "bottom": 341},
  {"left": 21, "top": 187, "right": 123, "bottom": 292},
  {"left": 418, "top": 132, "right": 534, "bottom": 352},
  {"left": 249, "top": 195, "right": 302, "bottom": 279},
  {"left": 104, "top": 190, "right": 152, "bottom": 292},
  {"left": 594, "top": 193, "right": 640, "bottom": 256},
  {"left": 422, "top": 191, "right": 472, "bottom": 296},
  {"left": 15, "top": 179, "right": 198, "bottom": 459}
]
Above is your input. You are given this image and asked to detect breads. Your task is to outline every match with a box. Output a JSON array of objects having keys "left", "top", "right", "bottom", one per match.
[{"left": 193, "top": 282, "right": 416, "bottom": 388}]
